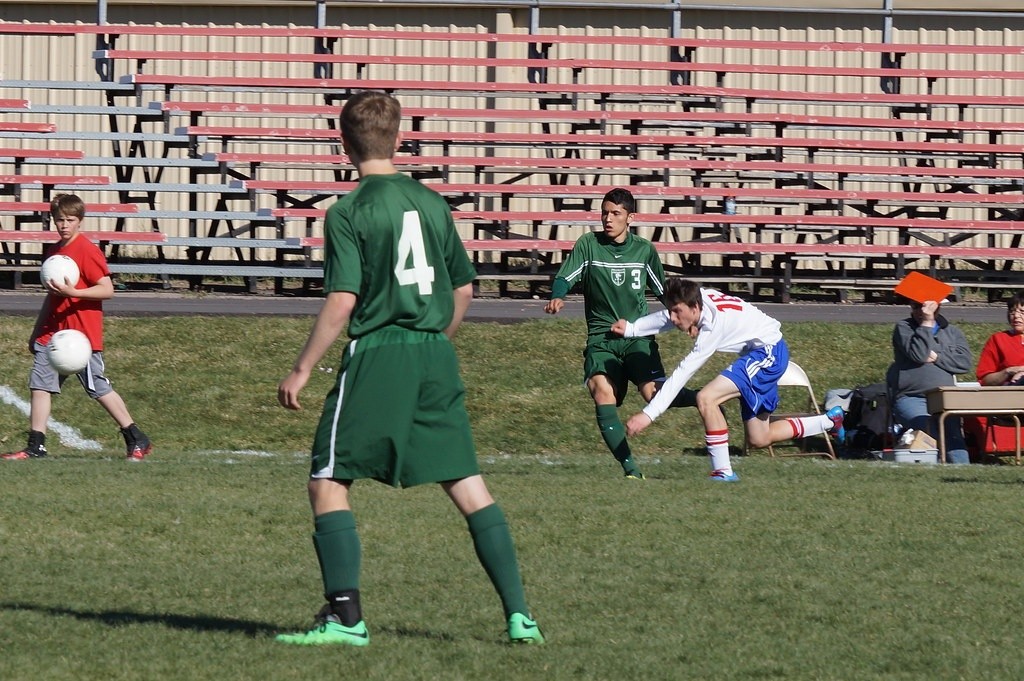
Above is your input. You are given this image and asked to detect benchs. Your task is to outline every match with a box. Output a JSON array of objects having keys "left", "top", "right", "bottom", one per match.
[{"left": 0, "top": 24, "right": 1024, "bottom": 311}]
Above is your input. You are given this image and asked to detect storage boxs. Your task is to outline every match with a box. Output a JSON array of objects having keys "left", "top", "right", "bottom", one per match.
[{"left": 883, "top": 448, "right": 938, "bottom": 463}]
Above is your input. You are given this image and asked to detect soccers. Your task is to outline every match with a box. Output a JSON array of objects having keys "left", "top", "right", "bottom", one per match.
[
  {"left": 47, "top": 328, "right": 92, "bottom": 374},
  {"left": 39, "top": 254, "right": 80, "bottom": 291}
]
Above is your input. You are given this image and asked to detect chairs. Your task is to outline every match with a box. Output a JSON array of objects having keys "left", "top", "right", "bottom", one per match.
[
  {"left": 891, "top": 358, "right": 964, "bottom": 442},
  {"left": 745, "top": 361, "right": 838, "bottom": 460}
]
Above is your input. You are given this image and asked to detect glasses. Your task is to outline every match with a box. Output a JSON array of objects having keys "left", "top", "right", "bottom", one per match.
[
  {"left": 1007, "top": 309, "right": 1024, "bottom": 315},
  {"left": 911, "top": 303, "right": 922, "bottom": 309}
]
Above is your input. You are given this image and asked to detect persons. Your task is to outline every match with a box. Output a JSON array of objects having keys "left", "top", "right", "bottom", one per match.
[
  {"left": 0, "top": 194, "right": 151, "bottom": 462},
  {"left": 885, "top": 298, "right": 971, "bottom": 464},
  {"left": 543, "top": 188, "right": 726, "bottom": 481},
  {"left": 976, "top": 292, "right": 1024, "bottom": 386},
  {"left": 610, "top": 275, "right": 847, "bottom": 482},
  {"left": 274, "top": 92, "right": 545, "bottom": 646}
]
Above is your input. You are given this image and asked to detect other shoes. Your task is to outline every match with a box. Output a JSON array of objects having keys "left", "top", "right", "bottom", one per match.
[
  {"left": 827, "top": 406, "right": 848, "bottom": 447},
  {"left": 623, "top": 469, "right": 646, "bottom": 482},
  {"left": 695, "top": 386, "right": 704, "bottom": 391},
  {"left": 707, "top": 468, "right": 739, "bottom": 483}
]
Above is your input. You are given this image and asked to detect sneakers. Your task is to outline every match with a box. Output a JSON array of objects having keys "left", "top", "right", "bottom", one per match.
[
  {"left": 1, "top": 448, "right": 47, "bottom": 460},
  {"left": 125, "top": 439, "right": 153, "bottom": 461},
  {"left": 499, "top": 610, "right": 547, "bottom": 647},
  {"left": 273, "top": 614, "right": 370, "bottom": 648}
]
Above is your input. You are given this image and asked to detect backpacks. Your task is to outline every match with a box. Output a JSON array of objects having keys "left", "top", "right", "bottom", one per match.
[{"left": 845, "top": 384, "right": 888, "bottom": 450}]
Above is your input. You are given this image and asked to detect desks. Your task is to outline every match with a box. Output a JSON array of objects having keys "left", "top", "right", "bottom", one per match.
[{"left": 922, "top": 386, "right": 1024, "bottom": 464}]
